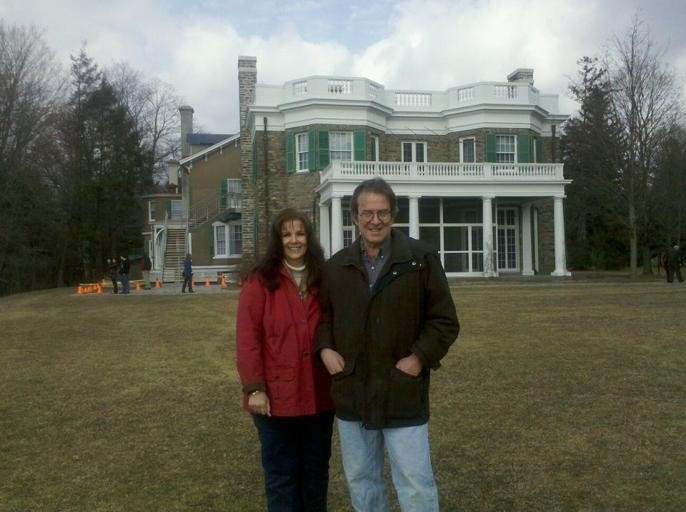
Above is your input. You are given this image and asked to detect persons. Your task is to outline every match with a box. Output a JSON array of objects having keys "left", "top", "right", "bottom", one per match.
[
  {"left": 106, "top": 253, "right": 130, "bottom": 294},
  {"left": 140, "top": 253, "right": 152, "bottom": 290},
  {"left": 181, "top": 253, "right": 194, "bottom": 293},
  {"left": 661, "top": 246, "right": 684, "bottom": 283}
]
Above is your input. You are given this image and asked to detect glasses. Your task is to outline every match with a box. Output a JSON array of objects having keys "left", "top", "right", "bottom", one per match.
[{"left": 356, "top": 210, "right": 393, "bottom": 219}]
